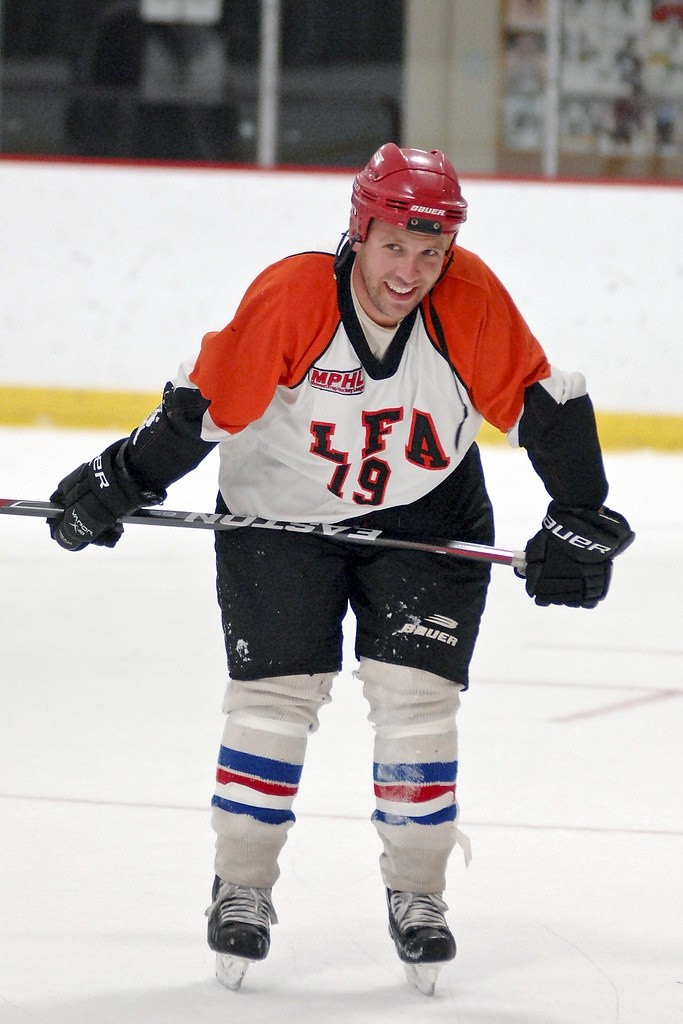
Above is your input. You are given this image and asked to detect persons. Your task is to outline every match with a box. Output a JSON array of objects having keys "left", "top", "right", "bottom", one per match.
[{"left": 46, "top": 142, "right": 636, "bottom": 995}]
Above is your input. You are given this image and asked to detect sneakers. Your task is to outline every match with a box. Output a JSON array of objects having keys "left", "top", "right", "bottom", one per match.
[
  {"left": 385, "top": 887, "right": 457, "bottom": 997},
  {"left": 204, "top": 874, "right": 279, "bottom": 991}
]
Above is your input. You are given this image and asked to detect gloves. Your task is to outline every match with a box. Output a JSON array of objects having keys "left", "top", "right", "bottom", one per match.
[
  {"left": 46, "top": 438, "right": 167, "bottom": 552},
  {"left": 514, "top": 502, "right": 636, "bottom": 610}
]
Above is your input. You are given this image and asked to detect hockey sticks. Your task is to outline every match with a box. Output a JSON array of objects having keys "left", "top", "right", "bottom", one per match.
[{"left": 0, "top": 497, "right": 534, "bottom": 578}]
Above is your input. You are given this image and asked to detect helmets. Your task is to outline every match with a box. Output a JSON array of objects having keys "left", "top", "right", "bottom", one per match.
[{"left": 350, "top": 143, "right": 468, "bottom": 258}]
